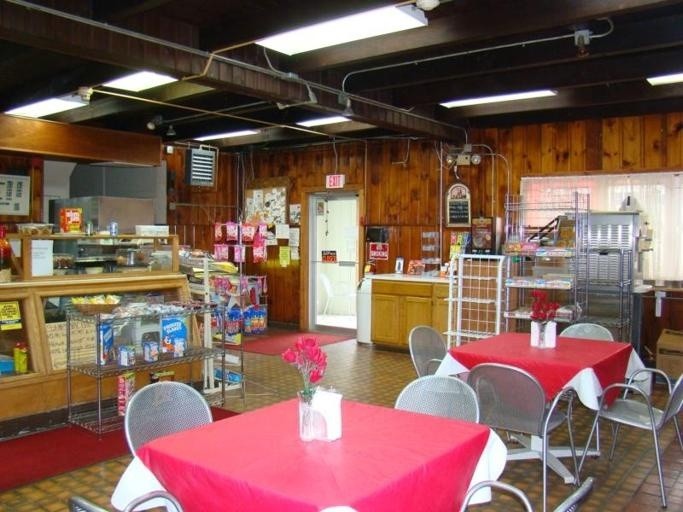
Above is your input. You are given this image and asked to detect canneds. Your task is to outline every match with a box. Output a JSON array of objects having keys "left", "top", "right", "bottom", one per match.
[
  {"left": 110, "top": 221, "right": 119, "bottom": 236},
  {"left": 85, "top": 222, "right": 95, "bottom": 236},
  {"left": 126, "top": 250, "right": 137, "bottom": 267}
]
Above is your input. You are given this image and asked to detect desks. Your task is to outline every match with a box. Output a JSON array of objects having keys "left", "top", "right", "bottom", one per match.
[
  {"left": 134, "top": 398, "right": 491, "bottom": 512},
  {"left": 448, "top": 331, "right": 633, "bottom": 486}
]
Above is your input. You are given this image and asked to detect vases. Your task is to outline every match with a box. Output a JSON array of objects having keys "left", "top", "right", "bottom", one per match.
[
  {"left": 537, "top": 323, "right": 548, "bottom": 351},
  {"left": 297, "top": 390, "right": 313, "bottom": 442}
]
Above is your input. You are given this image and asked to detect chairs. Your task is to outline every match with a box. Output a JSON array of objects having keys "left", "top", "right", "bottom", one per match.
[
  {"left": 66, "top": 488, "right": 188, "bottom": 512},
  {"left": 395, "top": 374, "right": 481, "bottom": 423},
  {"left": 407, "top": 324, "right": 446, "bottom": 378},
  {"left": 467, "top": 363, "right": 581, "bottom": 512},
  {"left": 124, "top": 381, "right": 214, "bottom": 456},
  {"left": 459, "top": 473, "right": 597, "bottom": 512},
  {"left": 578, "top": 367, "right": 683, "bottom": 508},
  {"left": 557, "top": 322, "right": 615, "bottom": 452}
]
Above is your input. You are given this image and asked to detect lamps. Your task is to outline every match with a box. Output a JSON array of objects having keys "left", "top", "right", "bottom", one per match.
[{"left": 253, "top": 3, "right": 430, "bottom": 58}]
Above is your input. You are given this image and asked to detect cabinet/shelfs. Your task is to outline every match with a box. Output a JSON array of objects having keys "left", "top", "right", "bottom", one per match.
[
  {"left": 0, "top": 232, "right": 205, "bottom": 444},
  {"left": 65, "top": 305, "right": 225, "bottom": 442},
  {"left": 505, "top": 191, "right": 653, "bottom": 356},
  {"left": 371, "top": 279, "right": 457, "bottom": 355},
  {"left": 443, "top": 260, "right": 518, "bottom": 350},
  {"left": 188, "top": 257, "right": 245, "bottom": 398}
]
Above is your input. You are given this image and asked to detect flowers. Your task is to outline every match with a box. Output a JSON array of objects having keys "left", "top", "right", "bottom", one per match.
[
  {"left": 528, "top": 289, "right": 560, "bottom": 323},
  {"left": 279, "top": 336, "right": 329, "bottom": 394}
]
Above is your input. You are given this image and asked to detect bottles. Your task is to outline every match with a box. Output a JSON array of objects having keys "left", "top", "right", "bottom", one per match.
[
  {"left": 85, "top": 221, "right": 93, "bottom": 236},
  {"left": 1, "top": 225, "right": 13, "bottom": 282},
  {"left": 14, "top": 342, "right": 30, "bottom": 375}
]
